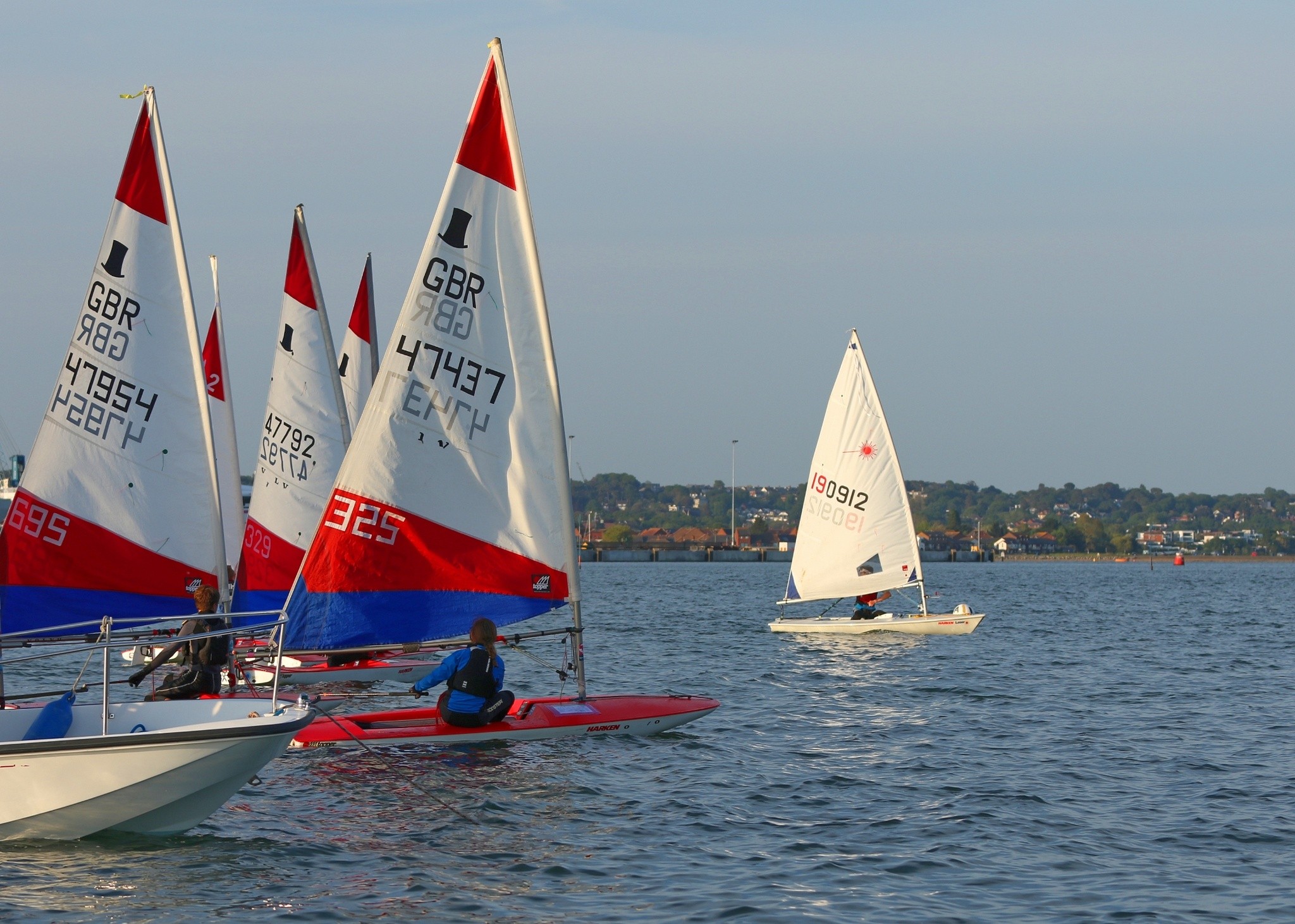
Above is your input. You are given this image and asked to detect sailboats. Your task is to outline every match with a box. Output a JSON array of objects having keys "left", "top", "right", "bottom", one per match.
[
  {"left": 767, "top": 328, "right": 987, "bottom": 636},
  {"left": 1, "top": 36, "right": 723, "bottom": 846}
]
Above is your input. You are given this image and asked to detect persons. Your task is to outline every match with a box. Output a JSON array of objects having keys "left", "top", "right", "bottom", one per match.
[
  {"left": 409, "top": 618, "right": 515, "bottom": 728},
  {"left": 851, "top": 565, "right": 892, "bottom": 620},
  {"left": 128, "top": 585, "right": 230, "bottom": 702}
]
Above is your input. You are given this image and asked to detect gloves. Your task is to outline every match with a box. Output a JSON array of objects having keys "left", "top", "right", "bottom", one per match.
[
  {"left": 409, "top": 685, "right": 422, "bottom": 699},
  {"left": 129, "top": 671, "right": 145, "bottom": 688}
]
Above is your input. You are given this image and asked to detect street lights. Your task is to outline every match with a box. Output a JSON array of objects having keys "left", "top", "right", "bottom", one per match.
[
  {"left": 731, "top": 439, "right": 739, "bottom": 546},
  {"left": 569, "top": 435, "right": 575, "bottom": 482}
]
